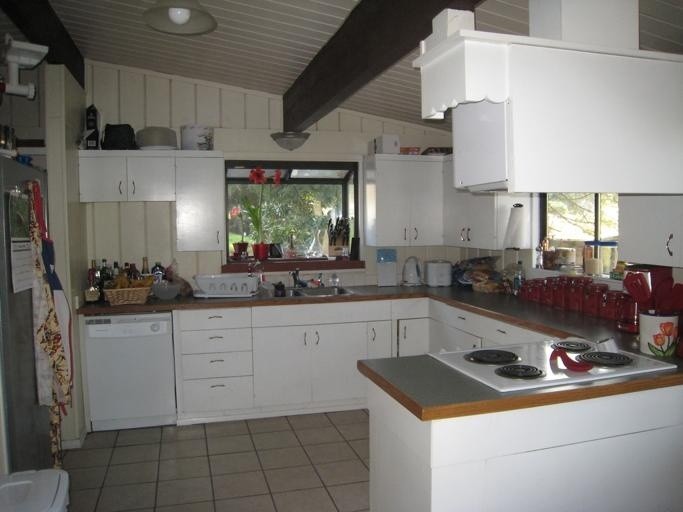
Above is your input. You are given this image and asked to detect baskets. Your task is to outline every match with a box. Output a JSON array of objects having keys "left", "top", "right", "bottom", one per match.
[{"left": 102, "top": 287, "right": 150, "bottom": 307}]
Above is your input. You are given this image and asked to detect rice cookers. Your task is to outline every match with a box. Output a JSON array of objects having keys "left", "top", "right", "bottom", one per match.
[{"left": 423, "top": 259, "right": 452, "bottom": 288}]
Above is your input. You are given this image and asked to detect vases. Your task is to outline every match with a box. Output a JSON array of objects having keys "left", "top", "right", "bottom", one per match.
[{"left": 232, "top": 242, "right": 283, "bottom": 260}]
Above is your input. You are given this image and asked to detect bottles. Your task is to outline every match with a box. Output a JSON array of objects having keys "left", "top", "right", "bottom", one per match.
[
  {"left": 86, "top": 256, "right": 167, "bottom": 287},
  {"left": 512, "top": 259, "right": 526, "bottom": 298}
]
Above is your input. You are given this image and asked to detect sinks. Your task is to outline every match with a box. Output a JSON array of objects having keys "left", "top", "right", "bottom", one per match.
[{"left": 289, "top": 286, "right": 356, "bottom": 298}]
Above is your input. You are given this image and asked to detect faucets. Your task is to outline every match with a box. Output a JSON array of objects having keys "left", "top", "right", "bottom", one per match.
[{"left": 288, "top": 267, "right": 306, "bottom": 288}]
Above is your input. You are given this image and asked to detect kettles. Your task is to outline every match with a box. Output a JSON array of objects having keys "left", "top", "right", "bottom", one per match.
[{"left": 400, "top": 255, "right": 422, "bottom": 288}]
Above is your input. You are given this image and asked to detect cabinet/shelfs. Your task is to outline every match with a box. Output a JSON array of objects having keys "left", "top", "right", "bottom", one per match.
[
  {"left": 362, "top": 161, "right": 443, "bottom": 247},
  {"left": 171, "top": 308, "right": 253, "bottom": 419},
  {"left": 443, "top": 161, "right": 531, "bottom": 251},
  {"left": 78, "top": 157, "right": 175, "bottom": 202},
  {"left": 618, "top": 194, "right": 682, "bottom": 266},
  {"left": 169, "top": 157, "right": 225, "bottom": 253},
  {"left": 429, "top": 300, "right": 542, "bottom": 355},
  {"left": 367, "top": 317, "right": 430, "bottom": 359},
  {"left": 252, "top": 322, "right": 367, "bottom": 409}
]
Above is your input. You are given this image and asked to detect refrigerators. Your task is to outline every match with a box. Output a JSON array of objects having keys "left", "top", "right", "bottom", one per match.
[{"left": 0, "top": 155, "right": 55, "bottom": 475}]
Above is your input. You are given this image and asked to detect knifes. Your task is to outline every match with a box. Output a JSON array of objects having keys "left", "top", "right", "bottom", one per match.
[{"left": 327, "top": 216, "right": 350, "bottom": 247}]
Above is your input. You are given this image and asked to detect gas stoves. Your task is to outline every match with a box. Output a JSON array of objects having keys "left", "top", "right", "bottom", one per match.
[{"left": 425, "top": 332, "right": 679, "bottom": 395}]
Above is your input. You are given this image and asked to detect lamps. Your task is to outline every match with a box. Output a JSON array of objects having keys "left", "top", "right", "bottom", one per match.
[{"left": 142, "top": 1, "right": 217, "bottom": 37}]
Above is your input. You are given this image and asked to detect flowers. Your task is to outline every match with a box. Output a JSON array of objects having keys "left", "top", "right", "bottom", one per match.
[{"left": 229, "top": 167, "right": 281, "bottom": 242}]
